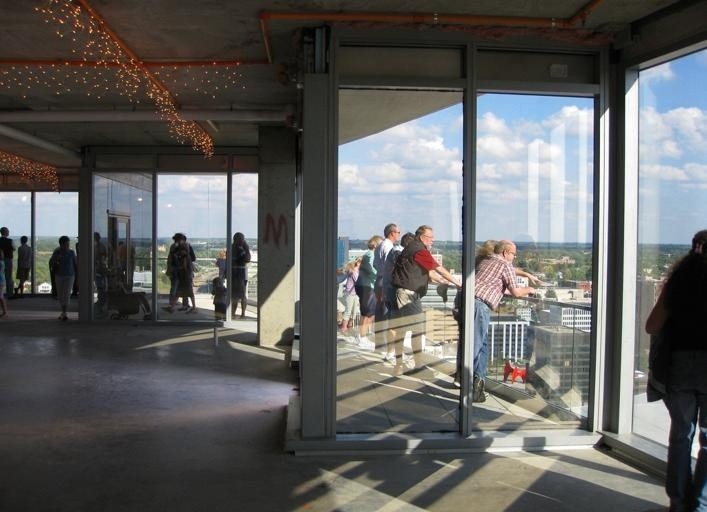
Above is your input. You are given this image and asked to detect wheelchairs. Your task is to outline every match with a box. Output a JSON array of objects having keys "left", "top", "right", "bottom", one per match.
[{"left": 102, "top": 266, "right": 152, "bottom": 321}]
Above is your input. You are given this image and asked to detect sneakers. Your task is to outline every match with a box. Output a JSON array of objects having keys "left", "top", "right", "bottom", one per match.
[
  {"left": 158, "top": 305, "right": 198, "bottom": 315},
  {"left": 394, "top": 363, "right": 410, "bottom": 375},
  {"left": 359, "top": 340, "right": 375, "bottom": 350},
  {"left": 414, "top": 366, "right": 440, "bottom": 379}
]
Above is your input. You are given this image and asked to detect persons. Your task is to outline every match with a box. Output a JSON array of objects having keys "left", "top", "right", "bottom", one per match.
[
  {"left": 164, "top": 233, "right": 198, "bottom": 313},
  {"left": 645, "top": 229, "right": 707, "bottom": 512},
  {"left": 48, "top": 232, "right": 135, "bottom": 321},
  {"left": 0, "top": 227, "right": 15, "bottom": 297},
  {"left": 14, "top": 236, "right": 31, "bottom": 296},
  {"left": 337, "top": 222, "right": 543, "bottom": 403},
  {"left": 212, "top": 277, "right": 229, "bottom": 322},
  {"left": 0, "top": 249, "right": 8, "bottom": 319},
  {"left": 215, "top": 249, "right": 227, "bottom": 283},
  {"left": 226, "top": 232, "right": 251, "bottom": 320}
]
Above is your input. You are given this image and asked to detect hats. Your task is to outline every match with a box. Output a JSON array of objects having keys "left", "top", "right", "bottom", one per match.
[
  {"left": 436, "top": 283, "right": 449, "bottom": 303},
  {"left": 172, "top": 233, "right": 187, "bottom": 239}
]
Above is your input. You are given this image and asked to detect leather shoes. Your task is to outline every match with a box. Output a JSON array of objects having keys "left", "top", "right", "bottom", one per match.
[{"left": 473, "top": 378, "right": 487, "bottom": 402}]
